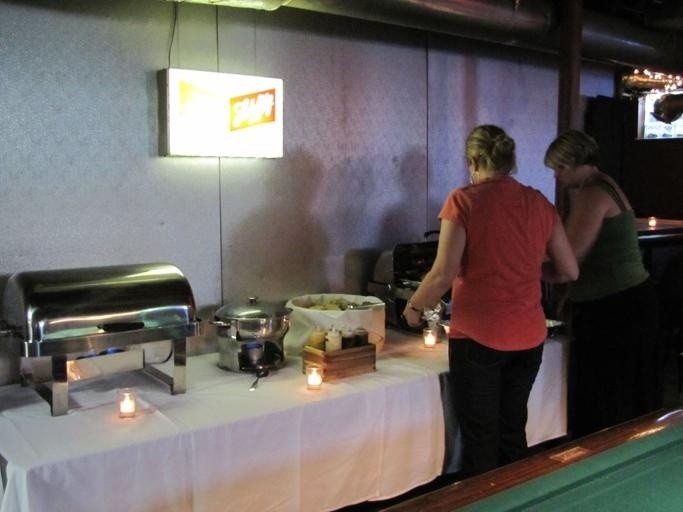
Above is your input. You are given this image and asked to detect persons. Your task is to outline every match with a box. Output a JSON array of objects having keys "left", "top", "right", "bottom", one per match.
[
  {"left": 542, "top": 129, "right": 650, "bottom": 442},
  {"left": 402, "top": 124, "right": 581, "bottom": 474}
]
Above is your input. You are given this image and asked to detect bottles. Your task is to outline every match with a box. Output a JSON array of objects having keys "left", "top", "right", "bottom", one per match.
[
  {"left": 324, "top": 326, "right": 342, "bottom": 353},
  {"left": 341, "top": 325, "right": 354, "bottom": 348},
  {"left": 354, "top": 322, "right": 368, "bottom": 347},
  {"left": 309, "top": 324, "right": 324, "bottom": 350}
]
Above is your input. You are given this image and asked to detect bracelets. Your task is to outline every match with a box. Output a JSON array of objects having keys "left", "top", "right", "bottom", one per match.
[{"left": 407, "top": 300, "right": 422, "bottom": 313}]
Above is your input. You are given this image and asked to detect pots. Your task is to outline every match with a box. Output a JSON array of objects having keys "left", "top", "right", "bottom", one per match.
[{"left": 208, "top": 295, "right": 298, "bottom": 342}]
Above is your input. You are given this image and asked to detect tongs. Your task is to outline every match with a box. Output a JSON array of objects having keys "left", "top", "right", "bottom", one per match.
[{"left": 344, "top": 301, "right": 385, "bottom": 310}]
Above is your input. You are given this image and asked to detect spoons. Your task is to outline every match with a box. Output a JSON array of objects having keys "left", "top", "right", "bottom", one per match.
[{"left": 249, "top": 368, "right": 269, "bottom": 391}]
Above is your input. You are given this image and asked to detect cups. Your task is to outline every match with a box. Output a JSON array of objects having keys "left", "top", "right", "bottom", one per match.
[
  {"left": 116, "top": 388, "right": 140, "bottom": 419},
  {"left": 422, "top": 326, "right": 437, "bottom": 351},
  {"left": 305, "top": 363, "right": 324, "bottom": 393}
]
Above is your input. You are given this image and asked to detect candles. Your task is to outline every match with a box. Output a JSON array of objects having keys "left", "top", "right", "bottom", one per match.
[
  {"left": 425, "top": 334, "right": 435, "bottom": 345},
  {"left": 120, "top": 396, "right": 135, "bottom": 413},
  {"left": 308, "top": 369, "right": 321, "bottom": 385}
]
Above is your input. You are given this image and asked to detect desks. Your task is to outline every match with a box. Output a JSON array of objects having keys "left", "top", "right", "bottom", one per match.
[{"left": 1, "top": 323, "right": 567, "bottom": 487}]
